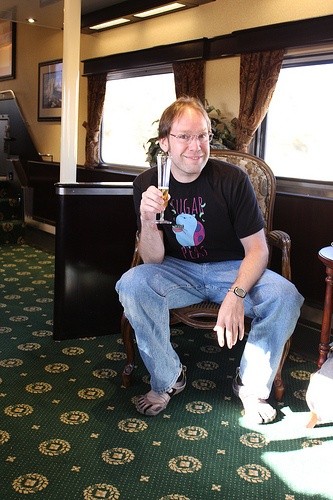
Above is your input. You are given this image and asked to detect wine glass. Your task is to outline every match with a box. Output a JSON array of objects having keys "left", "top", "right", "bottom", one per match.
[{"left": 152, "top": 156, "right": 173, "bottom": 224}]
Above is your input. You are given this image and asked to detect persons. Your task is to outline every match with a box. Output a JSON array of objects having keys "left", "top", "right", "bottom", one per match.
[{"left": 115, "top": 97, "right": 305, "bottom": 425}]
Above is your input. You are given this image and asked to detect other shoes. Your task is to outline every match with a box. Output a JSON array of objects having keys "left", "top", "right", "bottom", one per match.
[
  {"left": 136, "top": 369, "right": 186, "bottom": 416},
  {"left": 232, "top": 373, "right": 276, "bottom": 424}
]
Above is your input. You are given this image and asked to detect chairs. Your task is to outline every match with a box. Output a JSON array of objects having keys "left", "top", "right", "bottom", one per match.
[{"left": 122, "top": 150, "right": 292, "bottom": 399}]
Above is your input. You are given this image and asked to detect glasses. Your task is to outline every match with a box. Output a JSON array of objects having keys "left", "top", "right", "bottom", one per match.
[{"left": 169, "top": 133, "right": 214, "bottom": 142}]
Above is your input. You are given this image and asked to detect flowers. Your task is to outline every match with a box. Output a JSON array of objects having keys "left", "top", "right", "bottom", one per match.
[{"left": 143, "top": 100, "right": 235, "bottom": 170}]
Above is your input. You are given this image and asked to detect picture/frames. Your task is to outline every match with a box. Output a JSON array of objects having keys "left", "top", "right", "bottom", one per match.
[
  {"left": 0, "top": 6, "right": 17, "bottom": 83},
  {"left": 37, "top": 58, "right": 63, "bottom": 122}
]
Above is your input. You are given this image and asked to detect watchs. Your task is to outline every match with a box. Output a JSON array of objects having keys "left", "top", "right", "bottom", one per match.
[{"left": 228, "top": 286, "right": 248, "bottom": 299}]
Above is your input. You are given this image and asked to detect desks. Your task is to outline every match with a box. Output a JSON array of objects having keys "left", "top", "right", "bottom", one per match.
[{"left": 317, "top": 247, "right": 333, "bottom": 370}]
